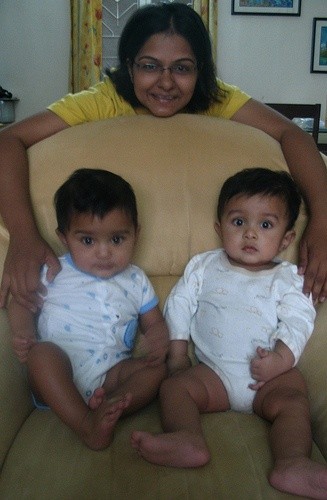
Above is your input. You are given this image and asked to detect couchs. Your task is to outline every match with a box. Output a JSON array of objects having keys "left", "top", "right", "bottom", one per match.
[{"left": 0, "top": 113, "right": 327, "bottom": 500}]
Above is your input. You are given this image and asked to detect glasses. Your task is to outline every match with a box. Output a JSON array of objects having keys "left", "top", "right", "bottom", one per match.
[{"left": 133, "top": 60, "right": 197, "bottom": 77}]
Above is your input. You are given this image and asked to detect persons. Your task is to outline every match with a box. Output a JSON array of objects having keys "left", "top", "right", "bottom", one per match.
[
  {"left": 128, "top": 166, "right": 327, "bottom": 499},
  {"left": 8, "top": 167, "right": 170, "bottom": 448},
  {"left": 0, "top": 3, "right": 327, "bottom": 315}
]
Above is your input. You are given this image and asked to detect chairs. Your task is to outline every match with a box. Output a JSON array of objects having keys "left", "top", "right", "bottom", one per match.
[{"left": 265, "top": 103, "right": 321, "bottom": 144}]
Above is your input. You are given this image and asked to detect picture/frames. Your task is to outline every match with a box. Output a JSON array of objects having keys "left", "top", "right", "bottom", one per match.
[
  {"left": 310, "top": 17, "right": 327, "bottom": 73},
  {"left": 230, "top": 0, "right": 301, "bottom": 16}
]
